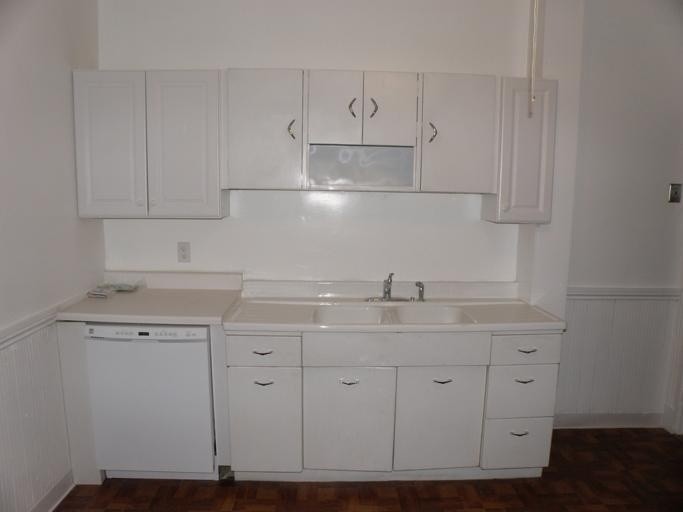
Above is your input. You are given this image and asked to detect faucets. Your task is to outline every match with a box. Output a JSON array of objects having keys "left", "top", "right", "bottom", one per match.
[
  {"left": 382, "top": 273, "right": 395, "bottom": 299},
  {"left": 416, "top": 281, "right": 425, "bottom": 299}
]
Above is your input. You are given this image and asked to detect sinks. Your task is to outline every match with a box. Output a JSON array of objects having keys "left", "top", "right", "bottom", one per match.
[
  {"left": 394, "top": 306, "right": 479, "bottom": 325},
  {"left": 312, "top": 306, "right": 393, "bottom": 324}
]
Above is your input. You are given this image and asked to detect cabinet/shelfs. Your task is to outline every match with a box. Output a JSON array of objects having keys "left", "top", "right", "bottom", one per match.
[
  {"left": 422, "top": 72, "right": 499, "bottom": 197},
  {"left": 480, "top": 74, "right": 554, "bottom": 228},
  {"left": 226, "top": 328, "right": 304, "bottom": 479},
  {"left": 483, "top": 329, "right": 561, "bottom": 479},
  {"left": 303, "top": 332, "right": 492, "bottom": 480},
  {"left": 73, "top": 65, "right": 223, "bottom": 220},
  {"left": 227, "top": 68, "right": 305, "bottom": 193},
  {"left": 307, "top": 70, "right": 419, "bottom": 192}
]
